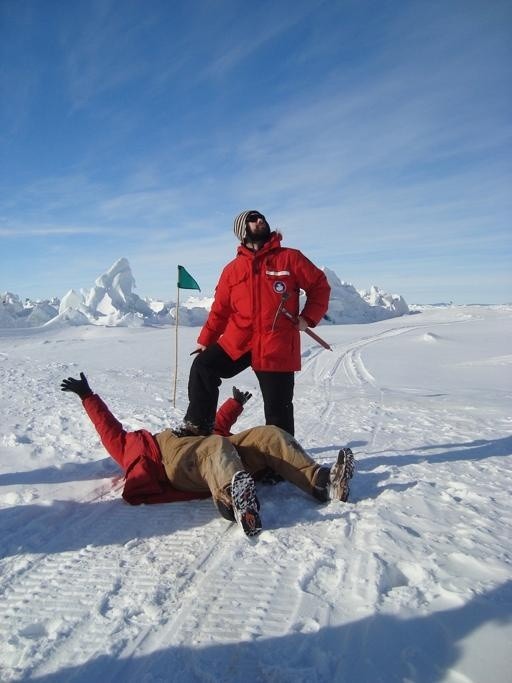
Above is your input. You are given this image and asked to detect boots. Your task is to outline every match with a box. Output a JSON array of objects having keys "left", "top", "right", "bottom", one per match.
[
  {"left": 313, "top": 448, "right": 356, "bottom": 502},
  {"left": 219, "top": 472, "right": 263, "bottom": 538}
]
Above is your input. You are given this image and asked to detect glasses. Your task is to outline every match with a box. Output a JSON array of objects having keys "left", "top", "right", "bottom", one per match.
[{"left": 246, "top": 215, "right": 265, "bottom": 222}]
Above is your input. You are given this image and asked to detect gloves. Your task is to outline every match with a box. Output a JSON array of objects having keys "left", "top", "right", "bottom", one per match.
[
  {"left": 60, "top": 372, "right": 93, "bottom": 400},
  {"left": 233, "top": 386, "right": 252, "bottom": 406}
]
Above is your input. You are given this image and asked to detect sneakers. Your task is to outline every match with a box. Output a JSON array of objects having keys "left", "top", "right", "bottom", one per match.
[
  {"left": 261, "top": 468, "right": 285, "bottom": 486},
  {"left": 172, "top": 422, "right": 216, "bottom": 438}
]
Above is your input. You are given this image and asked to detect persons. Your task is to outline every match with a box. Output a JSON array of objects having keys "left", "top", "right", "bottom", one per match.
[
  {"left": 178, "top": 210, "right": 331, "bottom": 438},
  {"left": 62, "top": 373, "right": 355, "bottom": 538}
]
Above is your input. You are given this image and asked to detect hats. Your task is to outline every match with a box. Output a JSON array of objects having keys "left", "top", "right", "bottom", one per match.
[{"left": 234, "top": 210, "right": 258, "bottom": 242}]
